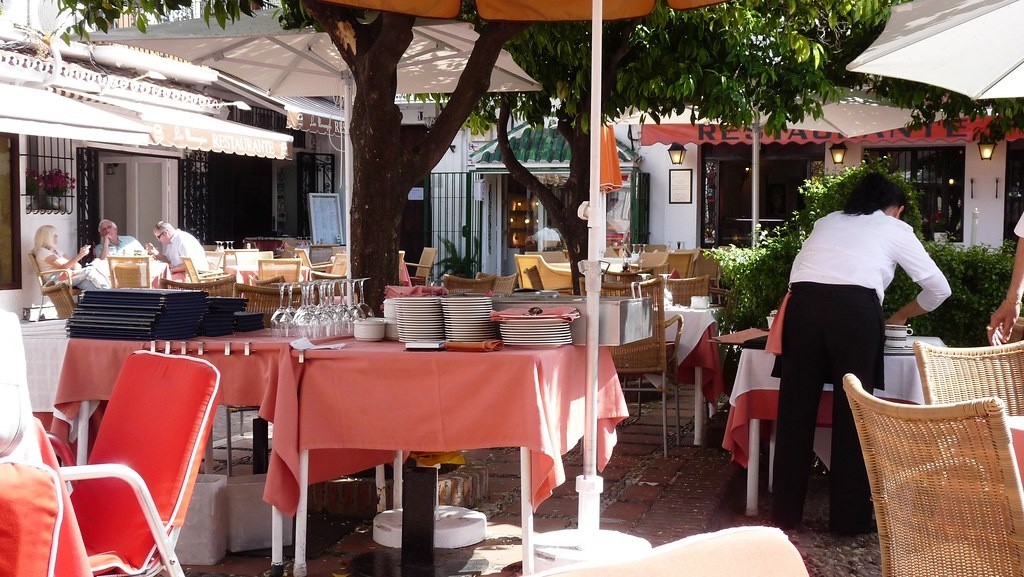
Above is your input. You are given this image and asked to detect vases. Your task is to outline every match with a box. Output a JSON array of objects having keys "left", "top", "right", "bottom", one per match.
[
  {"left": 27, "top": 196, "right": 34, "bottom": 208},
  {"left": 46, "top": 196, "right": 61, "bottom": 210}
]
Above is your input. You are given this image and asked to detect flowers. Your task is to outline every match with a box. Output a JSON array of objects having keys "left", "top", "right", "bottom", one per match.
[{"left": 25, "top": 169, "right": 75, "bottom": 197}]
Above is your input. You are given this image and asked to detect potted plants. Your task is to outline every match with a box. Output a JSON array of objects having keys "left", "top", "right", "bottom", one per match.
[{"left": 434, "top": 225, "right": 481, "bottom": 284}]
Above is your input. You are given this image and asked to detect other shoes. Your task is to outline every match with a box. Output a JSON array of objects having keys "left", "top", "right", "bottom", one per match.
[
  {"left": 773, "top": 512, "right": 803, "bottom": 530},
  {"left": 831, "top": 520, "right": 878, "bottom": 536}
]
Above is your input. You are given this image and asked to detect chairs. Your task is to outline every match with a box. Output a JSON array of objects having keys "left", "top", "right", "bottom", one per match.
[
  {"left": 399, "top": 245, "right": 719, "bottom": 458},
  {"left": 841, "top": 316, "right": 1024, "bottom": 576},
  {"left": 28, "top": 248, "right": 352, "bottom": 328},
  {"left": 0, "top": 350, "right": 221, "bottom": 577}
]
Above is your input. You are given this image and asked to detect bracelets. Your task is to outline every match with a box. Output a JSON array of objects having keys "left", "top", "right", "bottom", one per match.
[{"left": 1003, "top": 299, "right": 1022, "bottom": 305}]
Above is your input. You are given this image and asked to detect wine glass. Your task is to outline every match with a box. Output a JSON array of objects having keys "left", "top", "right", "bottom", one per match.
[
  {"left": 296, "top": 237, "right": 312, "bottom": 246},
  {"left": 214, "top": 241, "right": 235, "bottom": 252},
  {"left": 621, "top": 244, "right": 647, "bottom": 261},
  {"left": 270, "top": 277, "right": 374, "bottom": 339}
]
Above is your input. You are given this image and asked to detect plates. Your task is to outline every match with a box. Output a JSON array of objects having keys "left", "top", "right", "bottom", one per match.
[{"left": 393, "top": 297, "right": 572, "bottom": 348}]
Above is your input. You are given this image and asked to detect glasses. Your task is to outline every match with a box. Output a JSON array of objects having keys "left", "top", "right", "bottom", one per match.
[{"left": 156, "top": 230, "right": 169, "bottom": 239}]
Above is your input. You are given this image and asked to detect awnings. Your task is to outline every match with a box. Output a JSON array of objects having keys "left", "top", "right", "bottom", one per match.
[
  {"left": 0, "top": 83, "right": 295, "bottom": 161},
  {"left": 638, "top": 103, "right": 1024, "bottom": 146},
  {"left": 214, "top": 71, "right": 346, "bottom": 138}
]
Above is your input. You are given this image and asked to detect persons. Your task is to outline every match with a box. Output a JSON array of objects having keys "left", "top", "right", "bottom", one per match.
[
  {"left": 35, "top": 225, "right": 118, "bottom": 292},
  {"left": 988, "top": 211, "right": 1024, "bottom": 346},
  {"left": 94, "top": 220, "right": 145, "bottom": 260},
  {"left": 145, "top": 220, "right": 209, "bottom": 288},
  {"left": 765, "top": 172, "right": 952, "bottom": 536},
  {"left": 534, "top": 218, "right": 561, "bottom": 250},
  {"left": 0, "top": 306, "right": 43, "bottom": 467}
]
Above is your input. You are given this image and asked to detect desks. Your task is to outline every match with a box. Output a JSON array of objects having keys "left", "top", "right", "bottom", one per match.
[
  {"left": 91, "top": 259, "right": 172, "bottom": 290},
  {"left": 222, "top": 265, "right": 312, "bottom": 286},
  {"left": 48, "top": 329, "right": 629, "bottom": 577},
  {"left": 205, "top": 250, "right": 274, "bottom": 265},
  {"left": 721, "top": 337, "right": 948, "bottom": 517},
  {"left": 664, "top": 306, "right": 726, "bottom": 447}
]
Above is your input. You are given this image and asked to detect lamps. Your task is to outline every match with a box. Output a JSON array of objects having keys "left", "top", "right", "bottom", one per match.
[
  {"left": 124, "top": 70, "right": 166, "bottom": 84},
  {"left": 829, "top": 142, "right": 848, "bottom": 164},
  {"left": 668, "top": 142, "right": 687, "bottom": 164},
  {"left": 204, "top": 101, "right": 252, "bottom": 111},
  {"left": 106, "top": 163, "right": 119, "bottom": 175},
  {"left": 977, "top": 138, "right": 998, "bottom": 160}
]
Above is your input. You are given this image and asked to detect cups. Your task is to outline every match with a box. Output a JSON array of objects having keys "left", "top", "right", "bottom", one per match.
[
  {"left": 691, "top": 295, "right": 710, "bottom": 308},
  {"left": 884, "top": 324, "right": 913, "bottom": 347},
  {"left": 353, "top": 299, "right": 398, "bottom": 341}
]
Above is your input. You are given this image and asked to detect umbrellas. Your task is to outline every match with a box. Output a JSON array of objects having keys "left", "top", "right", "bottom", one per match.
[
  {"left": 607, "top": 87, "right": 997, "bottom": 249},
  {"left": 845, "top": 0, "right": 1024, "bottom": 102},
  {"left": 61, "top": 0, "right": 543, "bottom": 306},
  {"left": 333, "top": 0, "right": 725, "bottom": 553},
  {"left": 599, "top": 114, "right": 625, "bottom": 253}
]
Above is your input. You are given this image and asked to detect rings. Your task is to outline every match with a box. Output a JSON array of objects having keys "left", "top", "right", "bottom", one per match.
[{"left": 986, "top": 324, "right": 996, "bottom": 330}]
